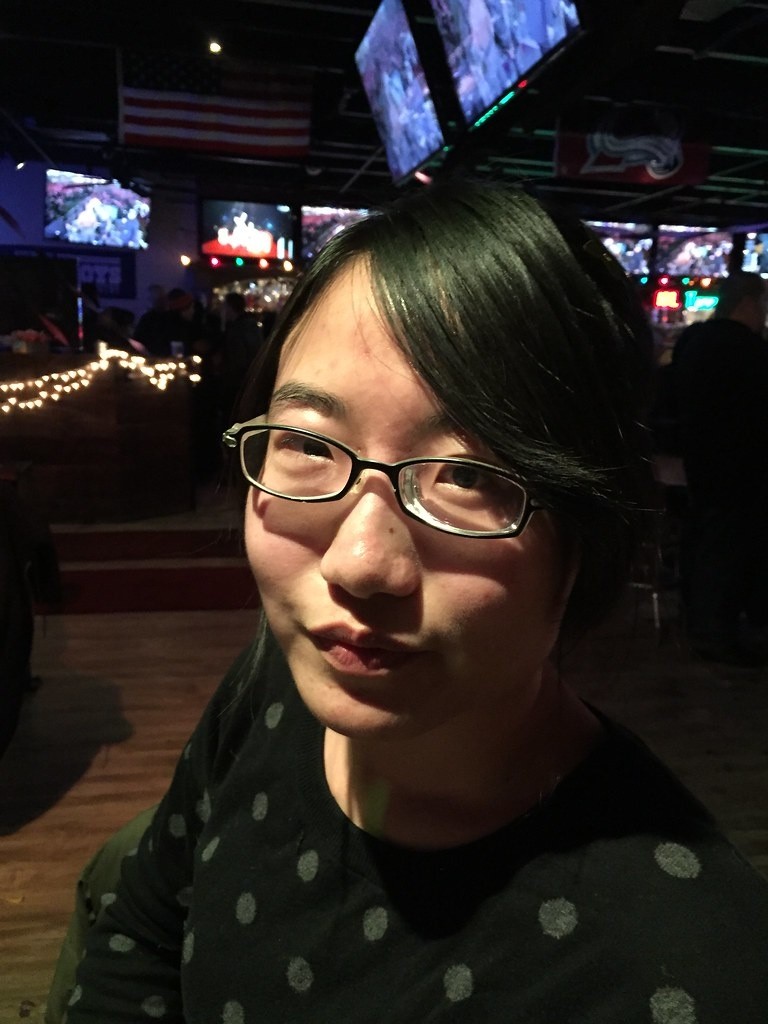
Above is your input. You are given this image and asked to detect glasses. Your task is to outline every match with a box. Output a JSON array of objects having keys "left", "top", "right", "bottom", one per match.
[{"left": 224, "top": 414, "right": 552, "bottom": 542}]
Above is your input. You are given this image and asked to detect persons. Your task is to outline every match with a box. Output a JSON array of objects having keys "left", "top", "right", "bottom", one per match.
[
  {"left": 63, "top": 177, "right": 768, "bottom": 1023},
  {"left": 33, "top": 256, "right": 263, "bottom": 486},
  {"left": 671, "top": 272, "right": 768, "bottom": 666}
]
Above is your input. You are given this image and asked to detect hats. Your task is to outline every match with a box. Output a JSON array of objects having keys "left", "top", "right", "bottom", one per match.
[{"left": 720, "top": 274, "right": 766, "bottom": 294}]
